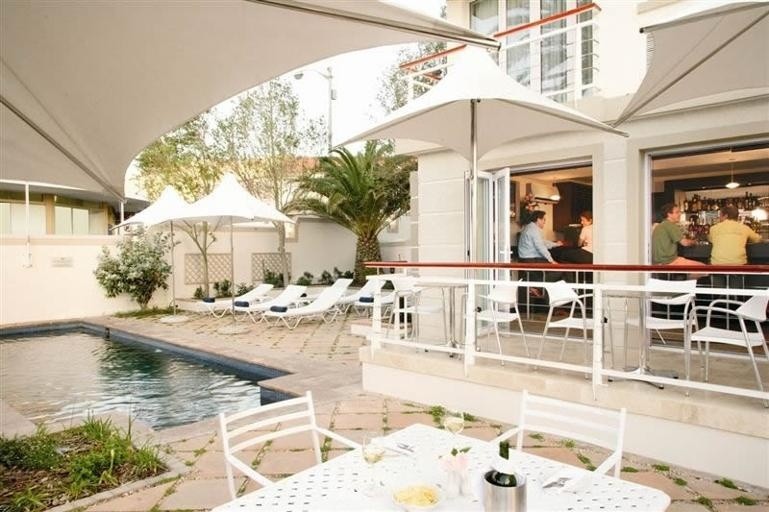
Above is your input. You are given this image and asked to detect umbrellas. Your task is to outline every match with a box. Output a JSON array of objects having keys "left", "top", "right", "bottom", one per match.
[
  {"left": 0, "top": 1, "right": 495, "bottom": 207},
  {"left": 613, "top": 4, "right": 768, "bottom": 127},
  {"left": 154, "top": 168, "right": 297, "bottom": 321},
  {"left": 110, "top": 182, "right": 194, "bottom": 315},
  {"left": 327, "top": 39, "right": 634, "bottom": 374}
]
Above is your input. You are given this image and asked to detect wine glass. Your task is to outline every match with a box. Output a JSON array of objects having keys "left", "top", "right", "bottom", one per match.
[
  {"left": 442, "top": 402, "right": 467, "bottom": 446},
  {"left": 360, "top": 431, "right": 386, "bottom": 477}
]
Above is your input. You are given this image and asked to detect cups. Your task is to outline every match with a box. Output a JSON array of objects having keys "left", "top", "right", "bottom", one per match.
[{"left": 482, "top": 471, "right": 526, "bottom": 511}]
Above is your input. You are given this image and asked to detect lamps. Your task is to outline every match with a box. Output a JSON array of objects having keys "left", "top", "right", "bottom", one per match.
[{"left": 726, "top": 164, "right": 740, "bottom": 189}]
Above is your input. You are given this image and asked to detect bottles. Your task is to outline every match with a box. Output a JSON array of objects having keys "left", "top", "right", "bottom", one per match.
[
  {"left": 682, "top": 189, "right": 761, "bottom": 234},
  {"left": 490, "top": 441, "right": 517, "bottom": 487}
]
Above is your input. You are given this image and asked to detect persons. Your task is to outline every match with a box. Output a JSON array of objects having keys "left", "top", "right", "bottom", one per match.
[
  {"left": 557, "top": 210, "right": 593, "bottom": 264},
  {"left": 516, "top": 209, "right": 575, "bottom": 317},
  {"left": 706, "top": 205, "right": 763, "bottom": 297},
  {"left": 651, "top": 202, "right": 708, "bottom": 285}
]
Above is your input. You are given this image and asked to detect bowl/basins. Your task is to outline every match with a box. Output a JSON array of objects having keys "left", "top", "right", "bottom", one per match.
[{"left": 392, "top": 484, "right": 445, "bottom": 510}]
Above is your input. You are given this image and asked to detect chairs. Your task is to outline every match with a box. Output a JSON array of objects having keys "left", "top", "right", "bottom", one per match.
[
  {"left": 625, "top": 279, "right": 706, "bottom": 386},
  {"left": 220, "top": 392, "right": 360, "bottom": 497},
  {"left": 195, "top": 272, "right": 406, "bottom": 338},
  {"left": 686, "top": 283, "right": 769, "bottom": 411},
  {"left": 531, "top": 280, "right": 611, "bottom": 381},
  {"left": 406, "top": 281, "right": 449, "bottom": 356},
  {"left": 462, "top": 280, "right": 533, "bottom": 369},
  {"left": 489, "top": 388, "right": 628, "bottom": 477}
]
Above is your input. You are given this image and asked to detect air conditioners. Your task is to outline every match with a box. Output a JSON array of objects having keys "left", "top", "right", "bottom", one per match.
[{"left": 526, "top": 183, "right": 562, "bottom": 205}]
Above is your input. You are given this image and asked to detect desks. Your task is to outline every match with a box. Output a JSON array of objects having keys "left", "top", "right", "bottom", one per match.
[
  {"left": 209, "top": 425, "right": 673, "bottom": 512},
  {"left": 415, "top": 280, "right": 482, "bottom": 359},
  {"left": 597, "top": 288, "right": 692, "bottom": 392}
]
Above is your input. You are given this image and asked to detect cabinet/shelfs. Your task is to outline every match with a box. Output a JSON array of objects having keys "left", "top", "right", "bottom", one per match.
[{"left": 664, "top": 172, "right": 769, "bottom": 245}]
